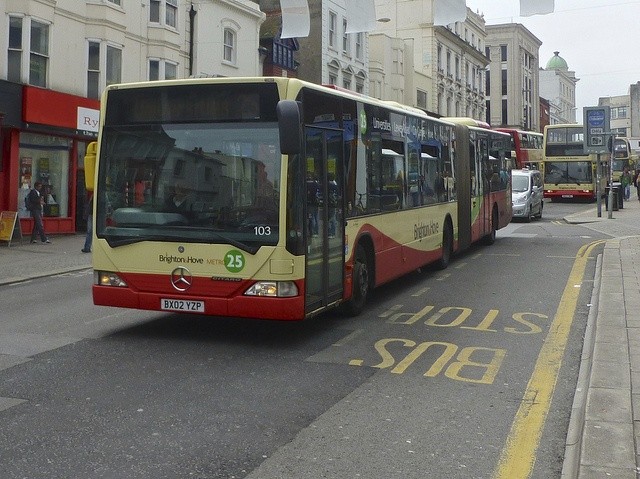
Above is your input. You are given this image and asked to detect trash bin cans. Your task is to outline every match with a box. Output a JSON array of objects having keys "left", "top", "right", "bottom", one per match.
[{"left": 608, "top": 181, "right": 623, "bottom": 209}]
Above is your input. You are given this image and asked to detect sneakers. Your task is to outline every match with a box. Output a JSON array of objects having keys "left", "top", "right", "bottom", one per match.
[
  {"left": 32, "top": 240, "right": 38, "bottom": 243},
  {"left": 43, "top": 240, "right": 54, "bottom": 245}
]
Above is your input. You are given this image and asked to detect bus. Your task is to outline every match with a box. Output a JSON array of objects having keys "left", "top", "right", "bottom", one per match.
[
  {"left": 613, "top": 137, "right": 631, "bottom": 187},
  {"left": 492, "top": 129, "right": 544, "bottom": 178},
  {"left": 84, "top": 77, "right": 512, "bottom": 320},
  {"left": 543, "top": 125, "right": 612, "bottom": 202}
]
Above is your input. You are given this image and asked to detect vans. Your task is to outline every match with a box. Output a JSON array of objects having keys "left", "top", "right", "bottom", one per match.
[{"left": 512, "top": 168, "right": 544, "bottom": 221}]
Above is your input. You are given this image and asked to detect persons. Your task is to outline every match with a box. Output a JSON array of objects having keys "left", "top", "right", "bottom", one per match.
[
  {"left": 444, "top": 171, "right": 454, "bottom": 202},
  {"left": 620, "top": 167, "right": 633, "bottom": 201},
  {"left": 43, "top": 184, "right": 56, "bottom": 204},
  {"left": 435, "top": 172, "right": 445, "bottom": 202},
  {"left": 166, "top": 180, "right": 192, "bottom": 213},
  {"left": 19, "top": 171, "right": 31, "bottom": 217},
  {"left": 25, "top": 182, "right": 51, "bottom": 243},
  {"left": 327, "top": 173, "right": 338, "bottom": 238},
  {"left": 81, "top": 192, "right": 92, "bottom": 253},
  {"left": 306, "top": 172, "right": 319, "bottom": 238},
  {"left": 266, "top": 175, "right": 300, "bottom": 222},
  {"left": 633, "top": 165, "right": 640, "bottom": 201},
  {"left": 471, "top": 169, "right": 475, "bottom": 195}
]
